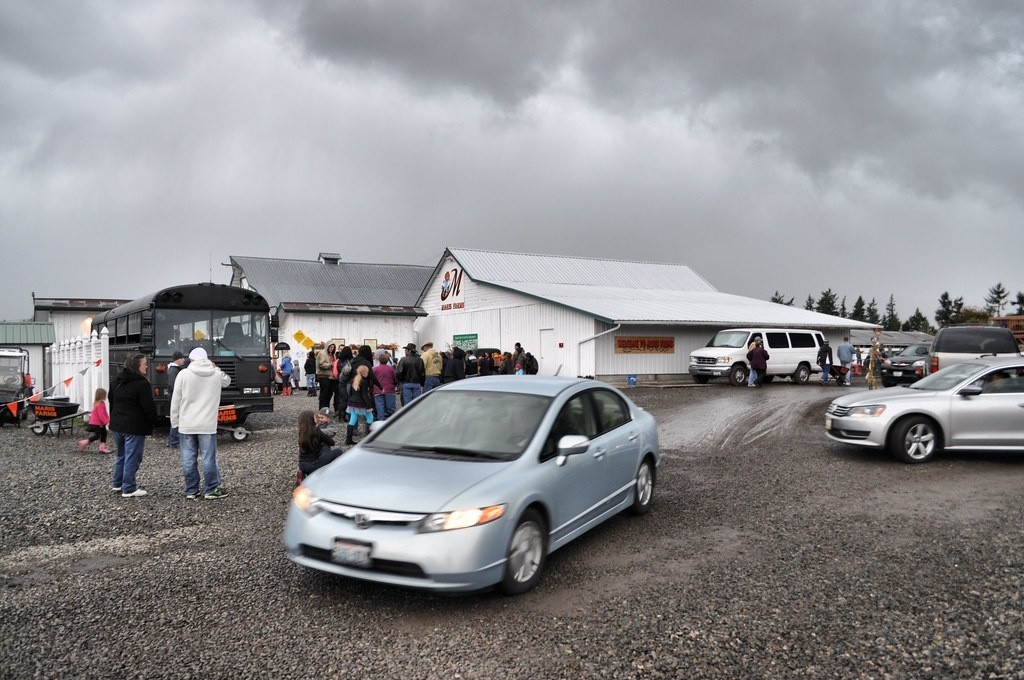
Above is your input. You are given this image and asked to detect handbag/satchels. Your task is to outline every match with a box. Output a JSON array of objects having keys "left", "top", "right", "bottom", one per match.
[{"left": 339, "top": 362, "right": 353, "bottom": 383}]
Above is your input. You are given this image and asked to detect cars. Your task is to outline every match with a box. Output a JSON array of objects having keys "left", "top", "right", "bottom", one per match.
[
  {"left": 285, "top": 375, "right": 661, "bottom": 597},
  {"left": 823, "top": 356, "right": 1024, "bottom": 465}
]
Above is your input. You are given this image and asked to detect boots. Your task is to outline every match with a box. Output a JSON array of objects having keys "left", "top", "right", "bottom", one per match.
[
  {"left": 307, "top": 387, "right": 313, "bottom": 397},
  {"left": 99, "top": 442, "right": 111, "bottom": 454},
  {"left": 365, "top": 423, "right": 371, "bottom": 435},
  {"left": 345, "top": 425, "right": 358, "bottom": 445},
  {"left": 78, "top": 439, "right": 90, "bottom": 452},
  {"left": 281, "top": 387, "right": 298, "bottom": 396},
  {"left": 312, "top": 386, "right": 317, "bottom": 396}
]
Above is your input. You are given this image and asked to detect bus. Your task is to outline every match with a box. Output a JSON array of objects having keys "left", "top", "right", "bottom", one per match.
[{"left": 89, "top": 282, "right": 280, "bottom": 429}]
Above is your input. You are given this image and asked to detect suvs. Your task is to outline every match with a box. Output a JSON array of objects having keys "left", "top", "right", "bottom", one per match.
[{"left": 880, "top": 342, "right": 934, "bottom": 388}]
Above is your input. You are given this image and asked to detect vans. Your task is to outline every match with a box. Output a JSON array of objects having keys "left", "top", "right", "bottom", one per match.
[
  {"left": 925, "top": 326, "right": 1021, "bottom": 378},
  {"left": 688, "top": 327, "right": 830, "bottom": 386}
]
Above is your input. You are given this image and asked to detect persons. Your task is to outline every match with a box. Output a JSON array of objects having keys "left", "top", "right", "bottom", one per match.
[
  {"left": 421, "top": 339, "right": 443, "bottom": 392},
  {"left": 272, "top": 354, "right": 300, "bottom": 396},
  {"left": 298, "top": 410, "right": 342, "bottom": 476},
  {"left": 108, "top": 354, "right": 156, "bottom": 497},
  {"left": 856, "top": 346, "right": 863, "bottom": 364},
  {"left": 515, "top": 363, "right": 524, "bottom": 376},
  {"left": 993, "top": 371, "right": 1010, "bottom": 392},
  {"left": 167, "top": 351, "right": 185, "bottom": 446},
  {"left": 304, "top": 340, "right": 398, "bottom": 445},
  {"left": 170, "top": 347, "right": 231, "bottom": 499},
  {"left": 747, "top": 337, "right": 769, "bottom": 388},
  {"left": 882, "top": 349, "right": 887, "bottom": 357},
  {"left": 26, "top": 373, "right": 31, "bottom": 386},
  {"left": 817, "top": 339, "right": 833, "bottom": 385},
  {"left": 77, "top": 388, "right": 111, "bottom": 453},
  {"left": 523, "top": 352, "right": 538, "bottom": 375},
  {"left": 837, "top": 337, "right": 856, "bottom": 385},
  {"left": 440, "top": 343, "right": 525, "bottom": 385},
  {"left": 397, "top": 343, "right": 426, "bottom": 405},
  {"left": 318, "top": 407, "right": 337, "bottom": 438}
]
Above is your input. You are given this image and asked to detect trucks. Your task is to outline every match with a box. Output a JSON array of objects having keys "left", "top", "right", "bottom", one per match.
[{"left": 0, "top": 346, "right": 32, "bottom": 423}]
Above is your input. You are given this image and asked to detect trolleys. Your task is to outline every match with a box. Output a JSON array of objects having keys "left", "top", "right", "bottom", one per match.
[{"left": 27, "top": 399, "right": 89, "bottom": 438}]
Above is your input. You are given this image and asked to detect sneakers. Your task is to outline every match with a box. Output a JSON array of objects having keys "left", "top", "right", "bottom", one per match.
[
  {"left": 113, "top": 485, "right": 122, "bottom": 492},
  {"left": 120, "top": 489, "right": 147, "bottom": 497},
  {"left": 204, "top": 489, "right": 228, "bottom": 500},
  {"left": 186, "top": 490, "right": 202, "bottom": 498}
]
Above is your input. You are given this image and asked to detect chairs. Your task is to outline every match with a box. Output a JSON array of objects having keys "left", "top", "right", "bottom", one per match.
[{"left": 221, "top": 321, "right": 244, "bottom": 342}]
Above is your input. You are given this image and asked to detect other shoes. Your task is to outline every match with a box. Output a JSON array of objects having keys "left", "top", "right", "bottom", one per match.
[
  {"left": 747, "top": 380, "right": 762, "bottom": 389},
  {"left": 819, "top": 379, "right": 824, "bottom": 386},
  {"left": 846, "top": 382, "right": 851, "bottom": 385}
]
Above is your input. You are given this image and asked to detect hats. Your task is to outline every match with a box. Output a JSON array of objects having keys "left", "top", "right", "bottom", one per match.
[
  {"left": 402, "top": 343, "right": 418, "bottom": 353},
  {"left": 754, "top": 337, "right": 763, "bottom": 342},
  {"left": 319, "top": 406, "right": 333, "bottom": 418},
  {"left": 421, "top": 342, "right": 433, "bottom": 350},
  {"left": 189, "top": 348, "right": 208, "bottom": 360},
  {"left": 173, "top": 351, "right": 189, "bottom": 361},
  {"left": 515, "top": 342, "right": 521, "bottom": 347}
]
